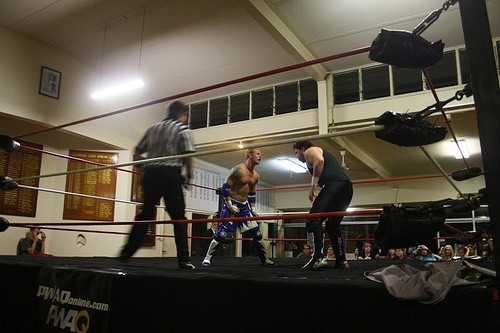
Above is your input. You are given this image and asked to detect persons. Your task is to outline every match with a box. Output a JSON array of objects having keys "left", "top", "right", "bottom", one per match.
[
  {"left": 293, "top": 141, "right": 353, "bottom": 270},
  {"left": 202, "top": 148, "right": 274, "bottom": 267},
  {"left": 296, "top": 237, "right": 493, "bottom": 260},
  {"left": 21, "top": 227, "right": 46, "bottom": 257},
  {"left": 118, "top": 100, "right": 196, "bottom": 270}
]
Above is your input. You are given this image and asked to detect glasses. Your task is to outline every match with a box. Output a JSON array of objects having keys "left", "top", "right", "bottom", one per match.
[{"left": 304, "top": 248, "right": 308, "bottom": 250}]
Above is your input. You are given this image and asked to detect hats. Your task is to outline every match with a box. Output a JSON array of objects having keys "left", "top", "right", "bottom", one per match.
[{"left": 418, "top": 245, "right": 428, "bottom": 250}]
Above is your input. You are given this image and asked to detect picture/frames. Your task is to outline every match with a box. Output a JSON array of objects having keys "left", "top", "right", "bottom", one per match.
[{"left": 38, "top": 66, "right": 62, "bottom": 99}]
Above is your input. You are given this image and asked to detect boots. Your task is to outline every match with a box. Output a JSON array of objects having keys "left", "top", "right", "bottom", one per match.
[
  {"left": 202, "top": 239, "right": 222, "bottom": 266},
  {"left": 253, "top": 239, "right": 274, "bottom": 265}
]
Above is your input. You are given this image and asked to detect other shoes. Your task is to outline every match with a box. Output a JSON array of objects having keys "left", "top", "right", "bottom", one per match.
[
  {"left": 300, "top": 258, "right": 328, "bottom": 270},
  {"left": 179, "top": 260, "right": 195, "bottom": 270},
  {"left": 336, "top": 261, "right": 349, "bottom": 268},
  {"left": 118, "top": 257, "right": 136, "bottom": 265}
]
